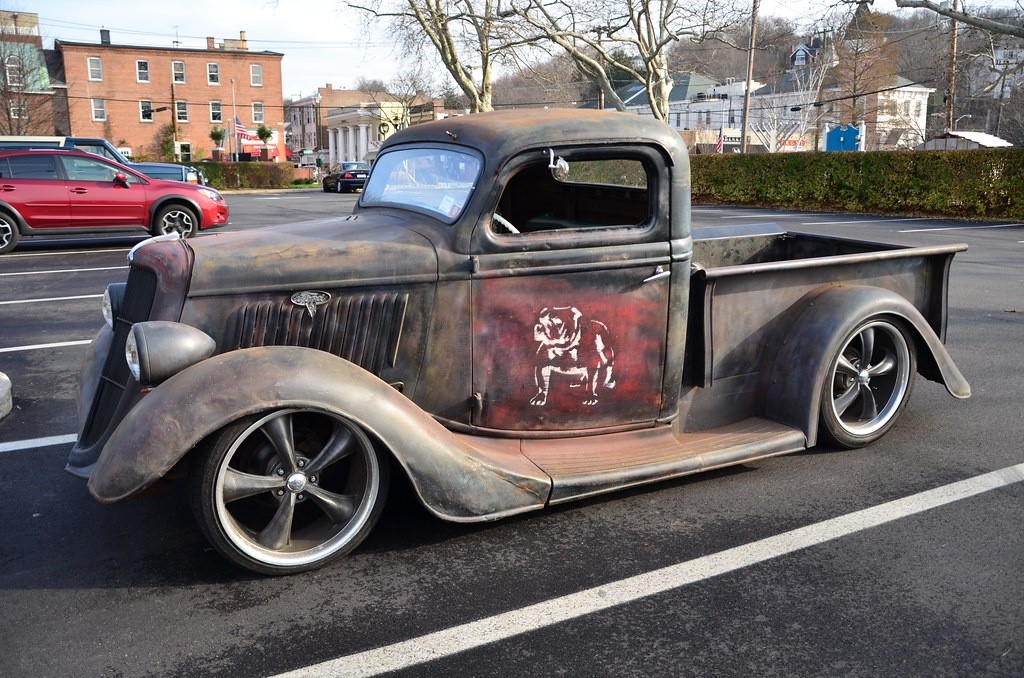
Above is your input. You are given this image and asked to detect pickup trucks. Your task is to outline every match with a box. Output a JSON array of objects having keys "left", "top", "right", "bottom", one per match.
[{"left": 61, "top": 103, "right": 972, "bottom": 576}]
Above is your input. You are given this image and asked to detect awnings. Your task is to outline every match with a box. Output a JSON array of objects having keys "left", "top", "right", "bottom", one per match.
[
  {"left": 285, "top": 145, "right": 292, "bottom": 156},
  {"left": 243, "top": 144, "right": 279, "bottom": 156}
]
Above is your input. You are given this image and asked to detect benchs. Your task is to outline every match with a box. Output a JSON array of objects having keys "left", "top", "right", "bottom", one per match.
[{"left": 525, "top": 217, "right": 602, "bottom": 231}]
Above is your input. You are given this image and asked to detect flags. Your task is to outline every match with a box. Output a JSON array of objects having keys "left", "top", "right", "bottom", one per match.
[
  {"left": 236, "top": 117, "right": 252, "bottom": 140},
  {"left": 716, "top": 134, "right": 722, "bottom": 153}
]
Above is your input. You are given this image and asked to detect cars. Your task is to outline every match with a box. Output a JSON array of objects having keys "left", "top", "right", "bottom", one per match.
[{"left": 322, "top": 161, "right": 372, "bottom": 194}]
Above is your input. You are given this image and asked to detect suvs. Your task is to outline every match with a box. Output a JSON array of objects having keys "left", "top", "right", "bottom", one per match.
[
  {"left": 0, "top": 145, "right": 230, "bottom": 255},
  {"left": 0, "top": 135, "right": 210, "bottom": 186}
]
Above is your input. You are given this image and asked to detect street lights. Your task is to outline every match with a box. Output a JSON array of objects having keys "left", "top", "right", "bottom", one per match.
[
  {"left": 955, "top": 114, "right": 973, "bottom": 132},
  {"left": 381, "top": 121, "right": 389, "bottom": 141},
  {"left": 393, "top": 115, "right": 401, "bottom": 132},
  {"left": 149, "top": 105, "right": 182, "bottom": 162}
]
